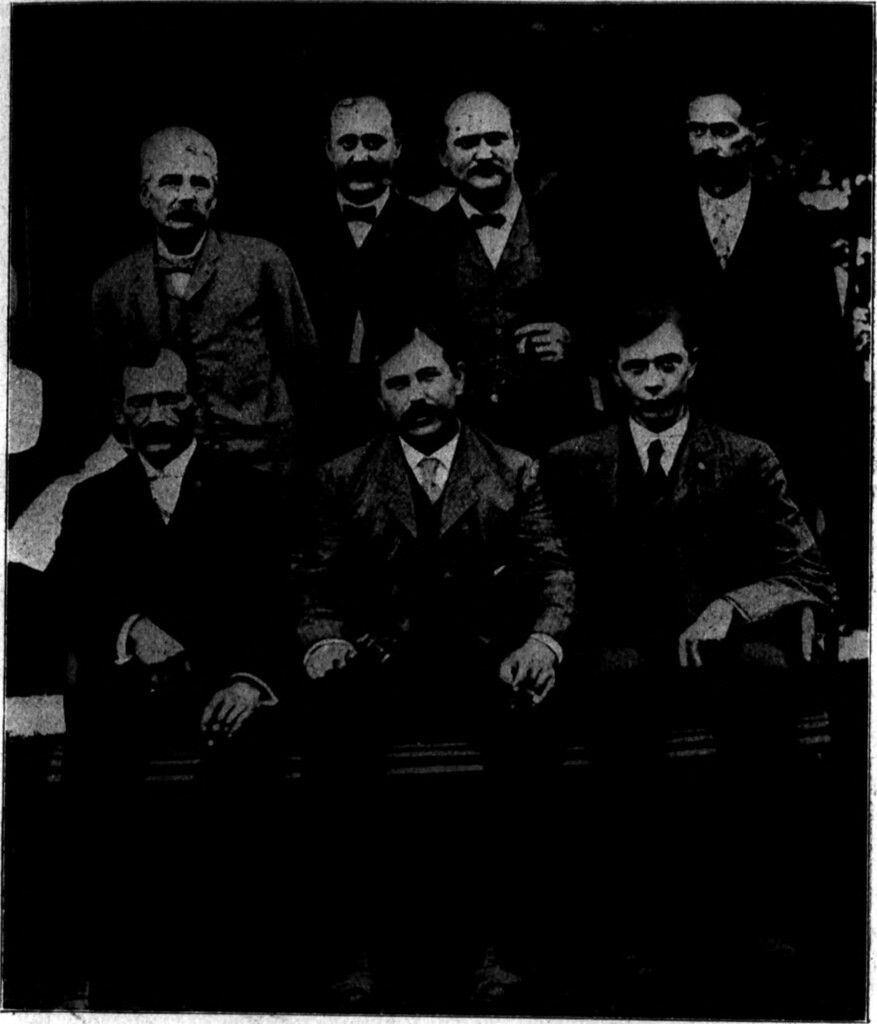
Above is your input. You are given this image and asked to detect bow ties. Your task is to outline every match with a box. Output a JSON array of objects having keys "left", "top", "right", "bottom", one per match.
[
  {"left": 158, "top": 255, "right": 197, "bottom": 275},
  {"left": 342, "top": 204, "right": 379, "bottom": 225},
  {"left": 467, "top": 213, "right": 509, "bottom": 231}
]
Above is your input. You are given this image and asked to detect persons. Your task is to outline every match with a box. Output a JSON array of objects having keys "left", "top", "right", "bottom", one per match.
[
  {"left": 274, "top": 87, "right": 862, "bottom": 472},
  {"left": 65, "top": 123, "right": 312, "bottom": 473},
  {"left": 531, "top": 295, "right": 834, "bottom": 724},
  {"left": 51, "top": 338, "right": 306, "bottom": 770},
  {"left": 290, "top": 307, "right": 575, "bottom": 743}
]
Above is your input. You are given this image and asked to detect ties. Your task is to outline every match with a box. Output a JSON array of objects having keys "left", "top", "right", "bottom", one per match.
[
  {"left": 644, "top": 439, "right": 668, "bottom": 505},
  {"left": 417, "top": 458, "right": 445, "bottom": 505},
  {"left": 710, "top": 206, "right": 732, "bottom": 269}
]
